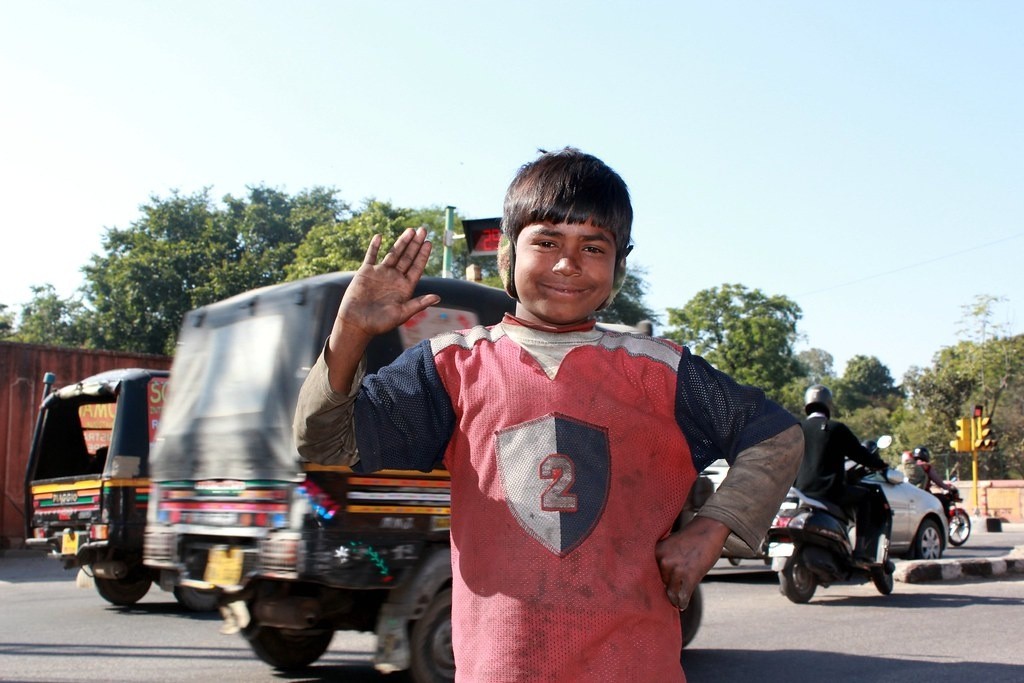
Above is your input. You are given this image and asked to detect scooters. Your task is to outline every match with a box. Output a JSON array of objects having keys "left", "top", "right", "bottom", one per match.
[{"left": 766, "top": 434, "right": 896, "bottom": 604}]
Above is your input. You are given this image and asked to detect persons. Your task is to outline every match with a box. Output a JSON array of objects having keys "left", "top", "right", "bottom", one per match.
[
  {"left": 900, "top": 447, "right": 952, "bottom": 493},
  {"left": 793, "top": 384, "right": 889, "bottom": 568},
  {"left": 291, "top": 148, "right": 805, "bottom": 683}
]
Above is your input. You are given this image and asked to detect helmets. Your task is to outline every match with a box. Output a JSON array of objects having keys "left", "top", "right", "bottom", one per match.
[
  {"left": 861, "top": 440, "right": 878, "bottom": 454},
  {"left": 805, "top": 384, "right": 834, "bottom": 421},
  {"left": 914, "top": 446, "right": 930, "bottom": 462}
]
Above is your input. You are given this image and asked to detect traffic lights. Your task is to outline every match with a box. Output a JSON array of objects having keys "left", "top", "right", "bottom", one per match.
[{"left": 972, "top": 405, "right": 991, "bottom": 450}]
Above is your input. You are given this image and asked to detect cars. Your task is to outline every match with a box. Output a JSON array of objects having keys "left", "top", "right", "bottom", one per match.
[{"left": 697, "top": 458, "right": 949, "bottom": 578}]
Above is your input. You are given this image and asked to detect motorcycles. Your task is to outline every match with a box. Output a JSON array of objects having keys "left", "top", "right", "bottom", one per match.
[
  {"left": 140, "top": 269, "right": 717, "bottom": 682},
  {"left": 923, "top": 475, "right": 972, "bottom": 546},
  {"left": 24, "top": 367, "right": 225, "bottom": 617}
]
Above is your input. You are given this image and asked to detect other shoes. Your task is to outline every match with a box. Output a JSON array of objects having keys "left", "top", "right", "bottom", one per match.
[{"left": 855, "top": 554, "right": 894, "bottom": 574}]
